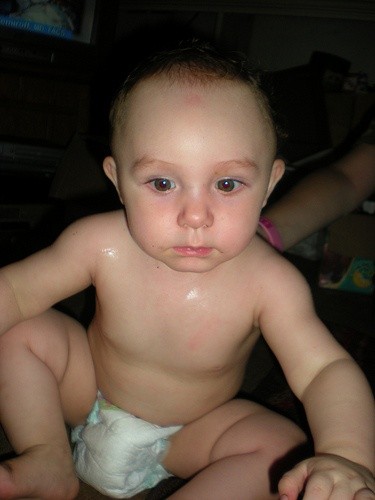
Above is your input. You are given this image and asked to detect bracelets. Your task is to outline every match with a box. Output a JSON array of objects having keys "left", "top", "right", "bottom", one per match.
[{"left": 257, "top": 214, "right": 287, "bottom": 255}]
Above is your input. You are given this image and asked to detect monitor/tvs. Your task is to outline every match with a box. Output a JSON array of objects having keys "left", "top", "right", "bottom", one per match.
[{"left": 0, "top": 0, "right": 116, "bottom": 66}]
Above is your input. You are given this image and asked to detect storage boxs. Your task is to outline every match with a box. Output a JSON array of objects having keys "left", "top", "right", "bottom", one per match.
[{"left": 307, "top": 49, "right": 375, "bottom": 296}]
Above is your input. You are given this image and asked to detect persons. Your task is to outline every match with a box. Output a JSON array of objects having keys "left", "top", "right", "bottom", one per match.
[
  {"left": 0, "top": 44, "right": 375, "bottom": 500},
  {"left": 258, "top": 122, "right": 374, "bottom": 254}
]
